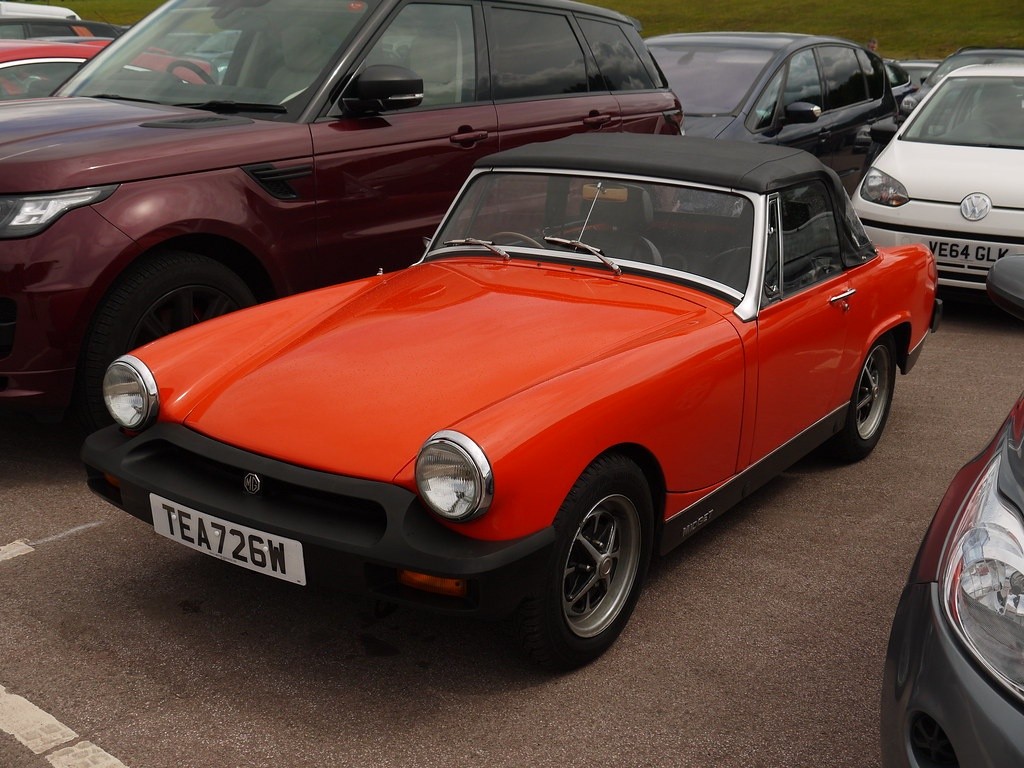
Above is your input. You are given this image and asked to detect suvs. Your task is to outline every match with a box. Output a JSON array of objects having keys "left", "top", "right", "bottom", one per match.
[{"left": 0, "top": 0, "right": 688, "bottom": 443}]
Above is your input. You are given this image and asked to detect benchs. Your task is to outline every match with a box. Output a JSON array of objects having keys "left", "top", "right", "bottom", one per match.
[{"left": 651, "top": 207, "right": 737, "bottom": 243}]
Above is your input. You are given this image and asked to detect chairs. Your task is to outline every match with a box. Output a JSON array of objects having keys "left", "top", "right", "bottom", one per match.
[
  {"left": 976, "top": 84, "right": 1018, "bottom": 119},
  {"left": 263, "top": 23, "right": 332, "bottom": 98},
  {"left": 402, "top": 35, "right": 458, "bottom": 95},
  {"left": 570, "top": 183, "right": 662, "bottom": 265},
  {"left": 719, "top": 200, "right": 815, "bottom": 274}
]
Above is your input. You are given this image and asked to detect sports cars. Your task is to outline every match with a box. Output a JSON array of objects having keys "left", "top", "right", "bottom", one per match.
[{"left": 77, "top": 116, "right": 947, "bottom": 673}]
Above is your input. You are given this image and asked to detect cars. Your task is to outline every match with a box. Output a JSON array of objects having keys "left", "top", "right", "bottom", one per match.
[
  {"left": 899, "top": 45, "right": 1024, "bottom": 134},
  {"left": 846, "top": 61, "right": 1024, "bottom": 293},
  {"left": 641, "top": 29, "right": 902, "bottom": 237},
  {"left": 0, "top": 1, "right": 238, "bottom": 103},
  {"left": 879, "top": 58, "right": 940, "bottom": 115},
  {"left": 881, "top": 252, "right": 1024, "bottom": 768}
]
